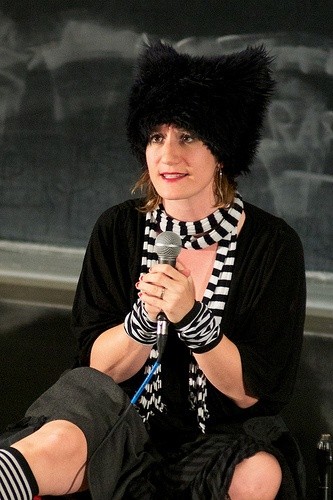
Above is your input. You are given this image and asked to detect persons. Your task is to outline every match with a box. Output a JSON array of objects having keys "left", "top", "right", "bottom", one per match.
[{"left": 0, "top": 37, "right": 309, "bottom": 500}]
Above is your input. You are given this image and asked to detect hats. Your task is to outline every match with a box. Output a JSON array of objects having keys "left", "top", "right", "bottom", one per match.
[{"left": 126, "top": 36, "right": 275, "bottom": 186}]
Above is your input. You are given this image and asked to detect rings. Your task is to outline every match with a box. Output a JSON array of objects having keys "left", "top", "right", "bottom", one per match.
[{"left": 160, "top": 287, "right": 166, "bottom": 300}]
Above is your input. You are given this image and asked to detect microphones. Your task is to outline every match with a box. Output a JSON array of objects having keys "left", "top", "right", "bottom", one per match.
[{"left": 153, "top": 230, "right": 183, "bottom": 355}]
